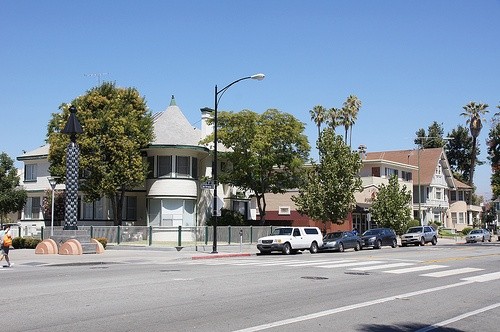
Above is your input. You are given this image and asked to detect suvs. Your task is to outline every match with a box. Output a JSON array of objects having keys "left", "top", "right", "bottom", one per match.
[
  {"left": 401, "top": 226, "right": 437, "bottom": 247},
  {"left": 256, "top": 226, "right": 324, "bottom": 255}
]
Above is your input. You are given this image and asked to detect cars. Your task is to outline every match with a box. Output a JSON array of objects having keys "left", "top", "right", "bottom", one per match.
[
  {"left": 465, "top": 229, "right": 491, "bottom": 243},
  {"left": 361, "top": 228, "right": 397, "bottom": 250},
  {"left": 321, "top": 230, "right": 363, "bottom": 253}
]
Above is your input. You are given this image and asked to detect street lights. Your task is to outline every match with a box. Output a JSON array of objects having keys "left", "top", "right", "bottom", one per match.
[
  {"left": 210, "top": 73, "right": 265, "bottom": 254},
  {"left": 418, "top": 139, "right": 434, "bottom": 226},
  {"left": 47, "top": 176, "right": 61, "bottom": 240}
]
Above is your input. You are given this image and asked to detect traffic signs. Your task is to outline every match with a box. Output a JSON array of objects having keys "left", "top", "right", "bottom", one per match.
[{"left": 200, "top": 184, "right": 214, "bottom": 189}]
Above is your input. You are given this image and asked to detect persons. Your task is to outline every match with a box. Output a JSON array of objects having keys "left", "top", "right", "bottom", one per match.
[{"left": 0, "top": 224, "right": 15, "bottom": 269}]
